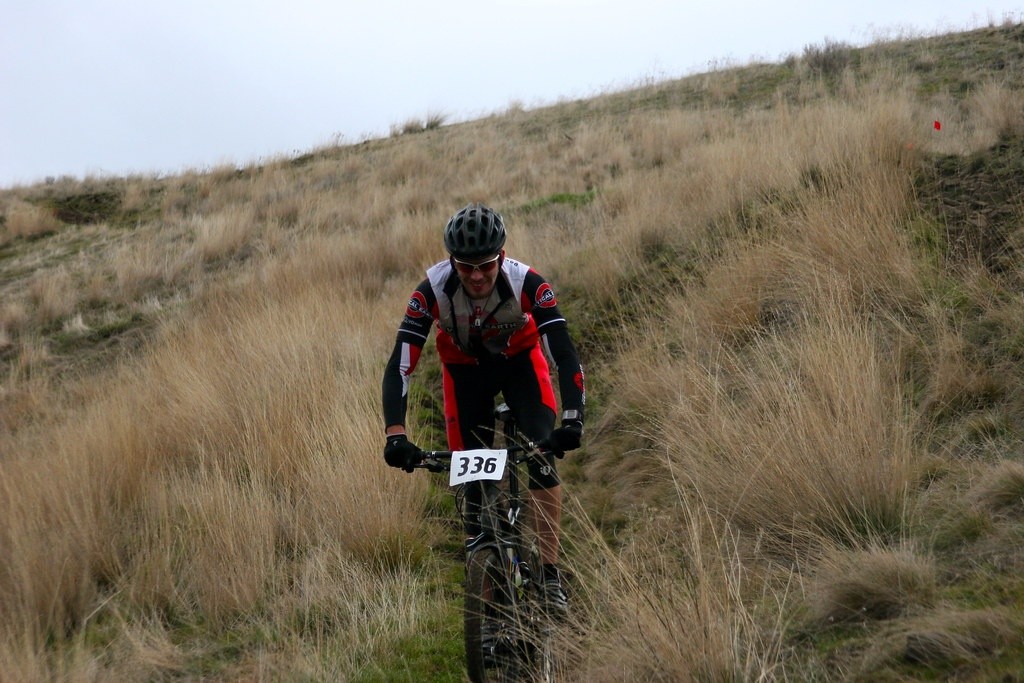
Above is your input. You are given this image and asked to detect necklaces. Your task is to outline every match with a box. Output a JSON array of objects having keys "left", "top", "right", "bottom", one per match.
[{"left": 462, "top": 285, "right": 495, "bottom": 327}]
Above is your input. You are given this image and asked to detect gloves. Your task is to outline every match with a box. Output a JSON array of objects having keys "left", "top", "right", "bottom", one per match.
[
  {"left": 549, "top": 420, "right": 584, "bottom": 459},
  {"left": 384, "top": 434, "right": 424, "bottom": 474}
]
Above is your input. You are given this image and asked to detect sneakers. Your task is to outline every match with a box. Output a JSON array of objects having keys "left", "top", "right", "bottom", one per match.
[
  {"left": 479, "top": 600, "right": 506, "bottom": 668},
  {"left": 537, "top": 564, "right": 569, "bottom": 619}
]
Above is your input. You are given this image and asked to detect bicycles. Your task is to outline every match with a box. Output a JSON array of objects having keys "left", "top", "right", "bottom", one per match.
[{"left": 400, "top": 401, "right": 573, "bottom": 682}]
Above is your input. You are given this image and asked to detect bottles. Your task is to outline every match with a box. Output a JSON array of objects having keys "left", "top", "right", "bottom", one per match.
[{"left": 506, "top": 547, "right": 525, "bottom": 599}]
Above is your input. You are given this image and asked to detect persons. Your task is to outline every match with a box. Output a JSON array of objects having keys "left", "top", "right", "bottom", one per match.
[{"left": 380, "top": 204, "right": 586, "bottom": 635}]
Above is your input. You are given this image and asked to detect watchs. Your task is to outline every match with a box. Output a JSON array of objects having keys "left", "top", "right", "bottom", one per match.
[{"left": 561, "top": 408, "right": 585, "bottom": 425}]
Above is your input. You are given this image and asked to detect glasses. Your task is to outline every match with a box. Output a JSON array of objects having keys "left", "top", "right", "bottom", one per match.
[{"left": 453, "top": 254, "right": 500, "bottom": 272}]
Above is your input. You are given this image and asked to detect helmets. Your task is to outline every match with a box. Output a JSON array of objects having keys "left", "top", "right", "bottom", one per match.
[{"left": 443, "top": 202, "right": 507, "bottom": 260}]
